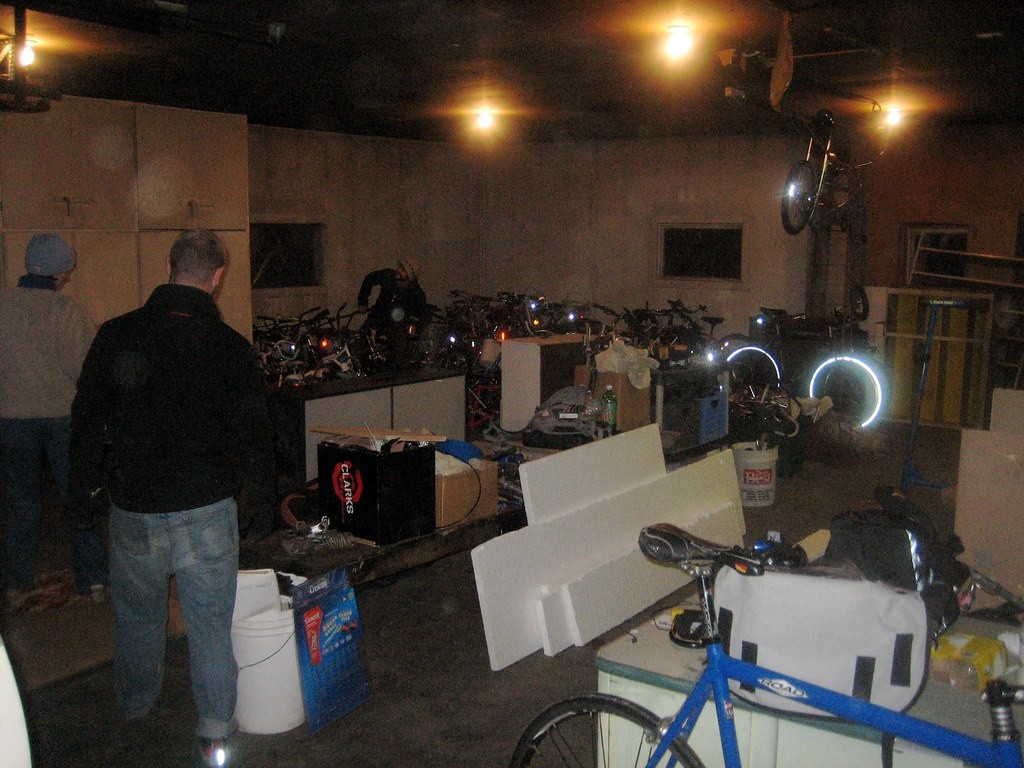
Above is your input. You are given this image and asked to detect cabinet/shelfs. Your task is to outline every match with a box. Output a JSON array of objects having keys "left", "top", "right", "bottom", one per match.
[
  {"left": 501, "top": 337, "right": 732, "bottom": 464},
  {"left": 856, "top": 225, "right": 1024, "bottom": 430},
  {"left": 597, "top": 588, "right": 1024, "bottom": 768},
  {"left": 279, "top": 366, "right": 467, "bottom": 489}
]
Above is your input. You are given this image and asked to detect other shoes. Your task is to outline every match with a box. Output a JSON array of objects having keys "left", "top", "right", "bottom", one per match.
[
  {"left": 199, "top": 736, "right": 230, "bottom": 768},
  {"left": 75, "top": 585, "right": 105, "bottom": 607}
]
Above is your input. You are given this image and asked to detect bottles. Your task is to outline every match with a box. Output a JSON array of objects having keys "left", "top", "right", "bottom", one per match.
[{"left": 600, "top": 385, "right": 617, "bottom": 428}]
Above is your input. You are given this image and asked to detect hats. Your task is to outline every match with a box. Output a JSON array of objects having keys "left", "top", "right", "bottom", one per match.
[
  {"left": 399, "top": 257, "right": 421, "bottom": 282},
  {"left": 25, "top": 234, "right": 75, "bottom": 276}
]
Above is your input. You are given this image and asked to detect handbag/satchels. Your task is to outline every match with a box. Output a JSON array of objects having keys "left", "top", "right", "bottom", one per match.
[
  {"left": 317, "top": 436, "right": 435, "bottom": 545},
  {"left": 828, "top": 500, "right": 959, "bottom": 644}
]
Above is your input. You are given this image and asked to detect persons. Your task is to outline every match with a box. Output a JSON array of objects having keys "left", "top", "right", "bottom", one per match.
[
  {"left": 356, "top": 257, "right": 427, "bottom": 339},
  {"left": 68, "top": 228, "right": 256, "bottom": 768},
  {"left": 0, "top": 235, "right": 108, "bottom": 594}
]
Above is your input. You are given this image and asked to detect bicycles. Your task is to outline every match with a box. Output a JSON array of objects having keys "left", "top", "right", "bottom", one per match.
[
  {"left": 778, "top": 108, "right": 869, "bottom": 246},
  {"left": 508, "top": 523, "right": 1024, "bottom": 768},
  {"left": 251, "top": 288, "right": 889, "bottom": 474}
]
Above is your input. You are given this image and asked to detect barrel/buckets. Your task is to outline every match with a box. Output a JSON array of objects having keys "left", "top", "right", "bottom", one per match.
[
  {"left": 230, "top": 595, "right": 309, "bottom": 736},
  {"left": 731, "top": 442, "right": 780, "bottom": 507}
]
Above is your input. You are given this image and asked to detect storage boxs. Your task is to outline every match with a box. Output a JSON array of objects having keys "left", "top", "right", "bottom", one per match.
[
  {"left": 576, "top": 364, "right": 653, "bottom": 430},
  {"left": 317, "top": 439, "right": 499, "bottom": 544}
]
